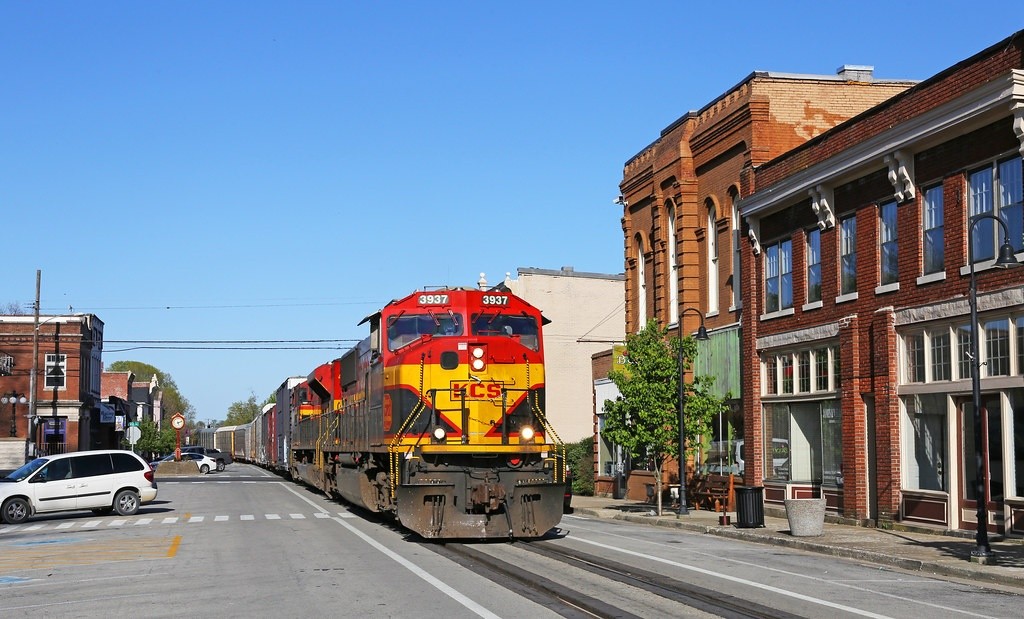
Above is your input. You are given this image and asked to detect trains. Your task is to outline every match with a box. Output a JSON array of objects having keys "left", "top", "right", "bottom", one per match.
[{"left": 177, "top": 279, "right": 575, "bottom": 543}]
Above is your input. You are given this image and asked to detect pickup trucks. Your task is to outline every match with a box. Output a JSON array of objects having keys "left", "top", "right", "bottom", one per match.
[{"left": 171, "top": 446, "right": 234, "bottom": 471}]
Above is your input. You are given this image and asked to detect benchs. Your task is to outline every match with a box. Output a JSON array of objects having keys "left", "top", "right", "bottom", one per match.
[{"left": 685, "top": 476, "right": 735, "bottom": 512}]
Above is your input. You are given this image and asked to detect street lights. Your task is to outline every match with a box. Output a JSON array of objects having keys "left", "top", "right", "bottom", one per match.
[
  {"left": 674, "top": 307, "right": 712, "bottom": 516},
  {"left": 963, "top": 211, "right": 1021, "bottom": 566}
]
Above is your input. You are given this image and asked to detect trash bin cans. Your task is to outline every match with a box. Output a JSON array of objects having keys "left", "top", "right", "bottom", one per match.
[{"left": 733, "top": 486, "right": 766, "bottom": 529}]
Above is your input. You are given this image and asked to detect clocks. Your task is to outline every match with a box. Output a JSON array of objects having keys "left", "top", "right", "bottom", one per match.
[{"left": 172, "top": 417, "right": 184, "bottom": 429}]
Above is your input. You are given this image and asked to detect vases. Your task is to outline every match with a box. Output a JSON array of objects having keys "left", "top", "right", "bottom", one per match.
[
  {"left": 643, "top": 482, "right": 665, "bottom": 504},
  {"left": 783, "top": 499, "right": 828, "bottom": 536},
  {"left": 668, "top": 484, "right": 681, "bottom": 501}
]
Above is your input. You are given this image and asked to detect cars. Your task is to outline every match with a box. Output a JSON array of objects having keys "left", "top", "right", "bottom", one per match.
[
  {"left": 0, "top": 449, "right": 160, "bottom": 524},
  {"left": 149, "top": 452, "right": 218, "bottom": 475}
]
